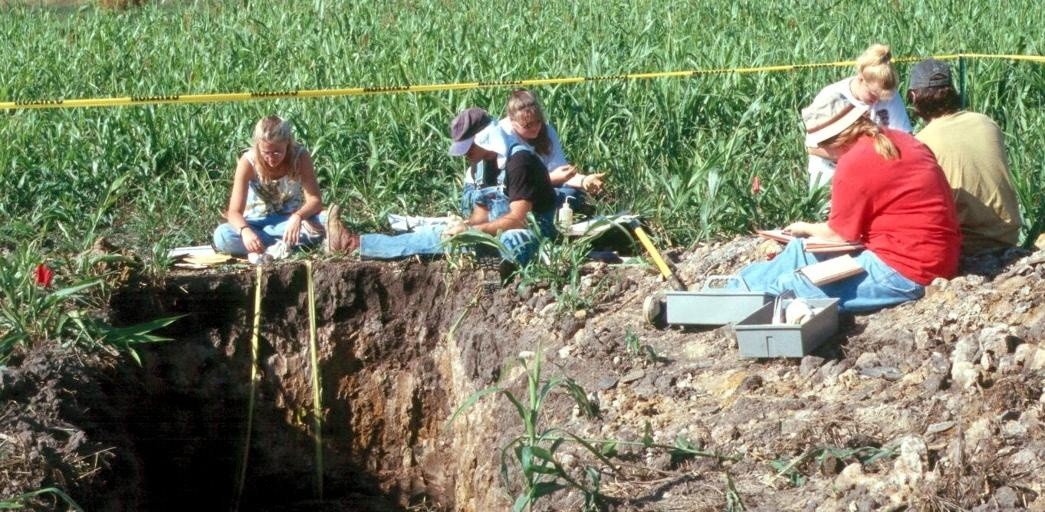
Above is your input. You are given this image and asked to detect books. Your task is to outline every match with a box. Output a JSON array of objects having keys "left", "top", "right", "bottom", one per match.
[{"left": 800, "top": 253, "right": 866, "bottom": 287}]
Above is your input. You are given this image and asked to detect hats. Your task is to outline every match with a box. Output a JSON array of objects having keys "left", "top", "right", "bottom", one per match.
[
  {"left": 448, "top": 107, "right": 513, "bottom": 167},
  {"left": 801, "top": 88, "right": 870, "bottom": 149},
  {"left": 906, "top": 58, "right": 952, "bottom": 96}
]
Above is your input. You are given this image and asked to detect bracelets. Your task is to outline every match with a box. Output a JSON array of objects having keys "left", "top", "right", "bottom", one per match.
[
  {"left": 294, "top": 212, "right": 304, "bottom": 220},
  {"left": 581, "top": 176, "right": 586, "bottom": 189},
  {"left": 237, "top": 226, "right": 250, "bottom": 239}
]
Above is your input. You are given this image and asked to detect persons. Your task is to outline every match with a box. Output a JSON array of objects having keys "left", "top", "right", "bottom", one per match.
[
  {"left": 326, "top": 107, "right": 559, "bottom": 295},
  {"left": 803, "top": 44, "right": 914, "bottom": 194},
  {"left": 902, "top": 57, "right": 1024, "bottom": 258},
  {"left": 461, "top": 89, "right": 607, "bottom": 229},
  {"left": 642, "top": 88, "right": 961, "bottom": 331},
  {"left": 213, "top": 115, "right": 326, "bottom": 266}
]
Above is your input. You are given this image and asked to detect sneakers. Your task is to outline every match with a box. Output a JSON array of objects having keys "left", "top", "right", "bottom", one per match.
[
  {"left": 325, "top": 202, "right": 350, "bottom": 255},
  {"left": 642, "top": 295, "right": 673, "bottom": 328}
]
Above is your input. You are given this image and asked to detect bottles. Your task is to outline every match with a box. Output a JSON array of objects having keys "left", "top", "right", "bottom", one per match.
[{"left": 558, "top": 202, "right": 574, "bottom": 228}]
